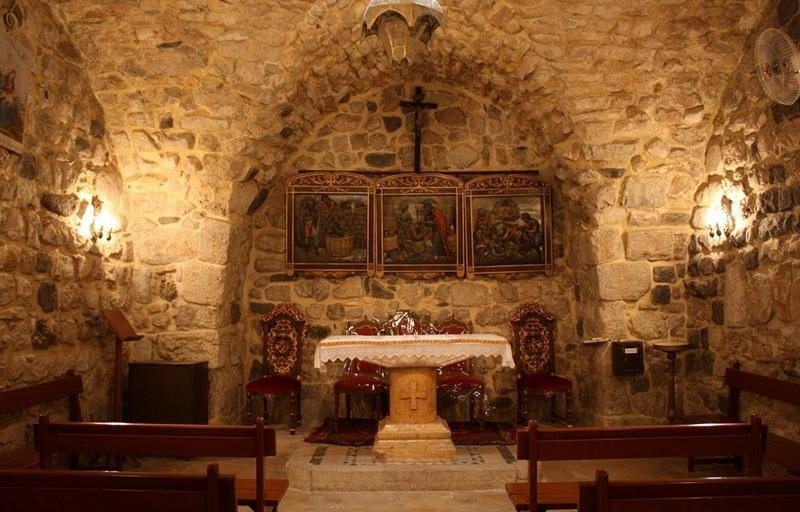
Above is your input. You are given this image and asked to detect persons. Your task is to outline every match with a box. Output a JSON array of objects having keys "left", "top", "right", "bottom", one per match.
[{"left": 301, "top": 194, "right": 545, "bottom": 265}]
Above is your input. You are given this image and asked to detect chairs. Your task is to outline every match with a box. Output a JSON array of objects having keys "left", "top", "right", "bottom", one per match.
[
  {"left": 331, "top": 308, "right": 487, "bottom": 427},
  {"left": 507, "top": 304, "right": 574, "bottom": 429},
  {"left": 244, "top": 302, "right": 309, "bottom": 433}
]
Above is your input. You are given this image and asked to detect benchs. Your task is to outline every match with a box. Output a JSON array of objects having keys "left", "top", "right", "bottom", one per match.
[
  {"left": 504, "top": 360, "right": 800, "bottom": 512},
  {"left": 0, "top": 369, "right": 290, "bottom": 512}
]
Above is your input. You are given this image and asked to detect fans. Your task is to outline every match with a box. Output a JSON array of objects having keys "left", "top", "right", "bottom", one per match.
[{"left": 755, "top": 27, "right": 800, "bottom": 106}]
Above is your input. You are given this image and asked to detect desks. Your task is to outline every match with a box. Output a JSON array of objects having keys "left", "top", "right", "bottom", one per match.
[{"left": 314, "top": 333, "right": 515, "bottom": 461}]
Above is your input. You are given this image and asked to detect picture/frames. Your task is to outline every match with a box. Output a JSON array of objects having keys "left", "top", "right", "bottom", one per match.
[
  {"left": 284, "top": 171, "right": 556, "bottom": 280},
  {"left": 0, "top": 27, "right": 33, "bottom": 155}
]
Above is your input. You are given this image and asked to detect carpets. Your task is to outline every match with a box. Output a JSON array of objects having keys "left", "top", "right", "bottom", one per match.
[{"left": 304, "top": 416, "right": 523, "bottom": 446}]
